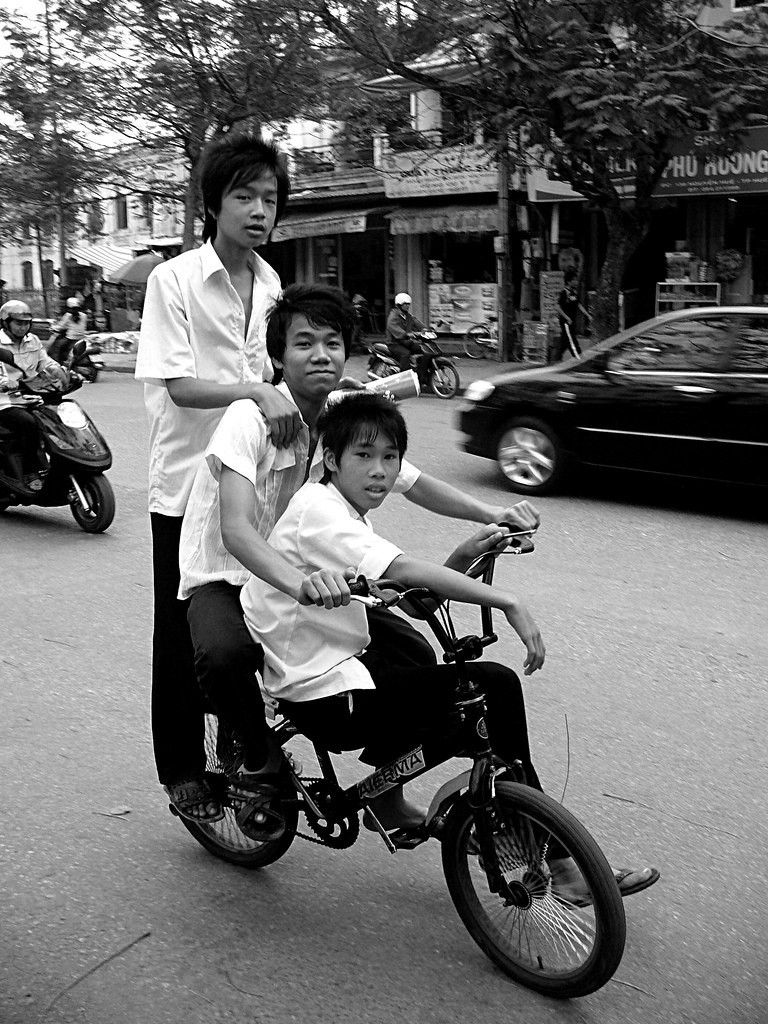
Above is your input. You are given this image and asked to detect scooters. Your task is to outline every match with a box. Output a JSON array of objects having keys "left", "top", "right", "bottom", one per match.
[
  {"left": 44, "top": 325, "right": 107, "bottom": 383},
  {"left": 0, "top": 341, "right": 117, "bottom": 534}
]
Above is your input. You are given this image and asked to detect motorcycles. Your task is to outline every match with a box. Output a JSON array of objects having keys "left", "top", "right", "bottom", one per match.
[{"left": 363, "top": 327, "right": 461, "bottom": 402}]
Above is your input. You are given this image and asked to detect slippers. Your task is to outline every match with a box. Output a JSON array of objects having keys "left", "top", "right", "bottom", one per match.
[
  {"left": 232, "top": 756, "right": 286, "bottom": 842},
  {"left": 561, "top": 868, "right": 661, "bottom": 910},
  {"left": 400, "top": 813, "right": 481, "bottom": 854},
  {"left": 163, "top": 773, "right": 225, "bottom": 824}
]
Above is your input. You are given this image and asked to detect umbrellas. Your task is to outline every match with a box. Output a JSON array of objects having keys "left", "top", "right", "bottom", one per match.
[{"left": 107, "top": 254, "right": 167, "bottom": 286}]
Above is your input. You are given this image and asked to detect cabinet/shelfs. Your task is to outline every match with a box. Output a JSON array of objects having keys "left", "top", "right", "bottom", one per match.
[{"left": 655, "top": 282, "right": 721, "bottom": 316}]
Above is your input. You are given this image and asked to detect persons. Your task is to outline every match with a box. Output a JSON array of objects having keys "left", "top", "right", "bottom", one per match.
[
  {"left": 0, "top": 300, "right": 75, "bottom": 492},
  {"left": 133, "top": 135, "right": 659, "bottom": 910},
  {"left": 555, "top": 270, "right": 594, "bottom": 364},
  {"left": 49, "top": 297, "right": 88, "bottom": 366},
  {"left": 385, "top": 293, "right": 425, "bottom": 372}
]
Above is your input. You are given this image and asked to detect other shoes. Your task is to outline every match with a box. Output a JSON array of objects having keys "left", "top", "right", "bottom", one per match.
[{"left": 26, "top": 478, "right": 43, "bottom": 491}]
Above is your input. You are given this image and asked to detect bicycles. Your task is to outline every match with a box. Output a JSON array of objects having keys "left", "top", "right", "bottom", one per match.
[
  {"left": 464, "top": 313, "right": 525, "bottom": 364},
  {"left": 166, "top": 526, "right": 630, "bottom": 1001}
]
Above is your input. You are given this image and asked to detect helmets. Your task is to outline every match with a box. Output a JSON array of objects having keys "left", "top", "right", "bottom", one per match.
[
  {"left": 67, "top": 297, "right": 80, "bottom": 307},
  {"left": 395, "top": 293, "right": 412, "bottom": 304},
  {"left": 0, "top": 300, "right": 32, "bottom": 322}
]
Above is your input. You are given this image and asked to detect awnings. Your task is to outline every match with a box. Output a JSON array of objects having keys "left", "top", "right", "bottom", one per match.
[
  {"left": 271, "top": 205, "right": 386, "bottom": 242},
  {"left": 383, "top": 201, "right": 532, "bottom": 235}
]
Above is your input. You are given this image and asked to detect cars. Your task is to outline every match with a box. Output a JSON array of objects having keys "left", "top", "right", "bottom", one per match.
[{"left": 453, "top": 305, "right": 768, "bottom": 513}]
